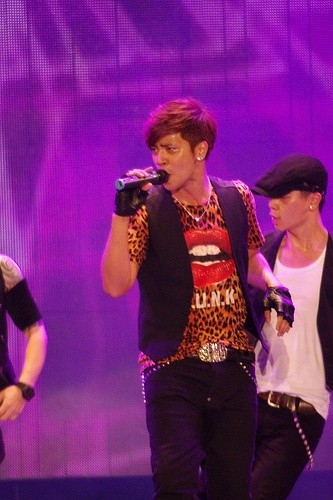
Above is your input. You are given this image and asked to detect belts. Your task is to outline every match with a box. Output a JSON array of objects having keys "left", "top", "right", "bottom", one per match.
[
  {"left": 257, "top": 391, "right": 319, "bottom": 420},
  {"left": 187, "top": 342, "right": 257, "bottom": 363}
]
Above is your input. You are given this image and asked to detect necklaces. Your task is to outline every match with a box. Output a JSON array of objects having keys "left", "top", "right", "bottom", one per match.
[{"left": 172, "top": 175, "right": 211, "bottom": 222}]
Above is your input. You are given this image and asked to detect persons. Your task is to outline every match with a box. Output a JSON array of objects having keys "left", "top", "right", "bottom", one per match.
[
  {"left": 0, "top": 254, "right": 48, "bottom": 465},
  {"left": 101, "top": 95, "right": 295, "bottom": 500},
  {"left": 249, "top": 154, "right": 333, "bottom": 500}
]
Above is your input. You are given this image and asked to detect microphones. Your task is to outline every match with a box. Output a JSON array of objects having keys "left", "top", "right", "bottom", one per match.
[{"left": 117, "top": 169, "right": 169, "bottom": 194}]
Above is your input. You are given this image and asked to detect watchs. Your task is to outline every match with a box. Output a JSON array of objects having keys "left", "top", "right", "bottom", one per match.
[{"left": 14, "top": 382, "right": 35, "bottom": 402}]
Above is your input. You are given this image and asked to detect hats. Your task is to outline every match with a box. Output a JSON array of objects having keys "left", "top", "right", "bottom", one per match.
[{"left": 250, "top": 154, "right": 328, "bottom": 198}]
